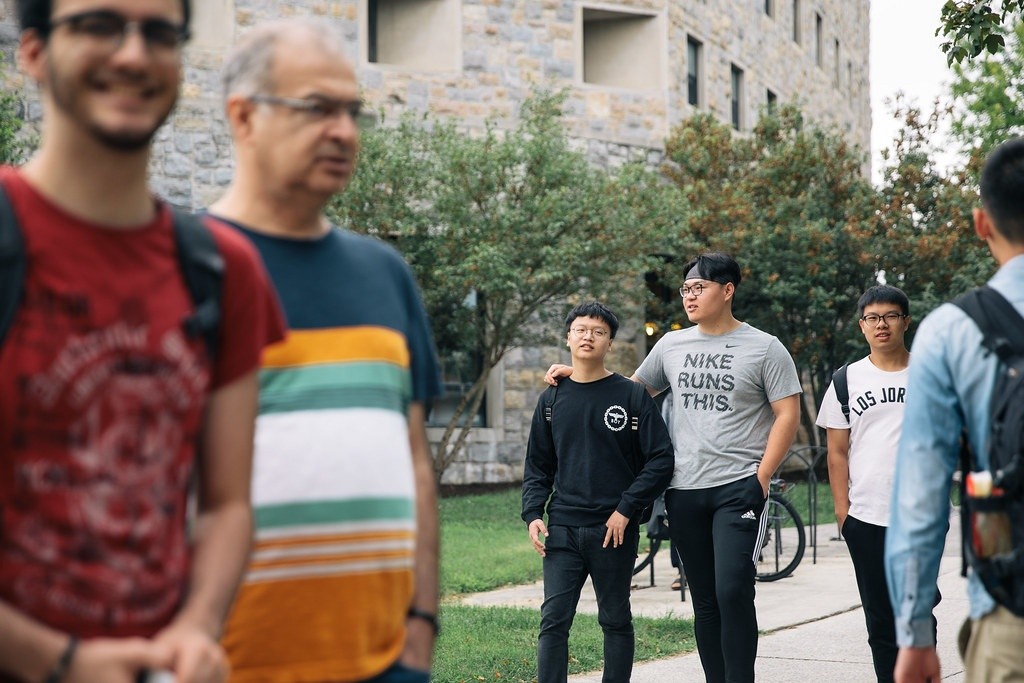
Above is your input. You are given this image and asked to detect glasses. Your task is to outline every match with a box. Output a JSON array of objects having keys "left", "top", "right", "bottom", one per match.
[
  {"left": 573, "top": 324, "right": 608, "bottom": 338},
  {"left": 246, "top": 95, "right": 364, "bottom": 117},
  {"left": 862, "top": 312, "right": 905, "bottom": 325},
  {"left": 679, "top": 282, "right": 703, "bottom": 298},
  {"left": 51, "top": 10, "right": 191, "bottom": 49}
]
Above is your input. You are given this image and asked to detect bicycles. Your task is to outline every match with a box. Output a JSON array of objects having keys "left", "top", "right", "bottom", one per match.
[{"left": 632, "top": 478, "right": 806, "bottom": 602}]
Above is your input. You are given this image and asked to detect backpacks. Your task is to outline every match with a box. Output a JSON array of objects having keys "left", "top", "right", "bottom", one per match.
[{"left": 945, "top": 281, "right": 1024, "bottom": 614}]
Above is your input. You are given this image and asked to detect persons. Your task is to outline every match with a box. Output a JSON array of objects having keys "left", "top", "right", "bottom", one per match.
[
  {"left": 522, "top": 303, "right": 675, "bottom": 683},
  {"left": 660, "top": 392, "right": 689, "bottom": 590},
  {"left": 1, "top": 0, "right": 292, "bottom": 683},
  {"left": 883, "top": 138, "right": 1024, "bottom": 682},
  {"left": 184, "top": 22, "right": 446, "bottom": 683},
  {"left": 814, "top": 285, "right": 964, "bottom": 682},
  {"left": 542, "top": 252, "right": 805, "bottom": 683}
]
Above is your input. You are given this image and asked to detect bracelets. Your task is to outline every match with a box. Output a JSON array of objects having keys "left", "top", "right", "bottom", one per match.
[
  {"left": 42, "top": 633, "right": 78, "bottom": 683},
  {"left": 407, "top": 606, "right": 439, "bottom": 637}
]
